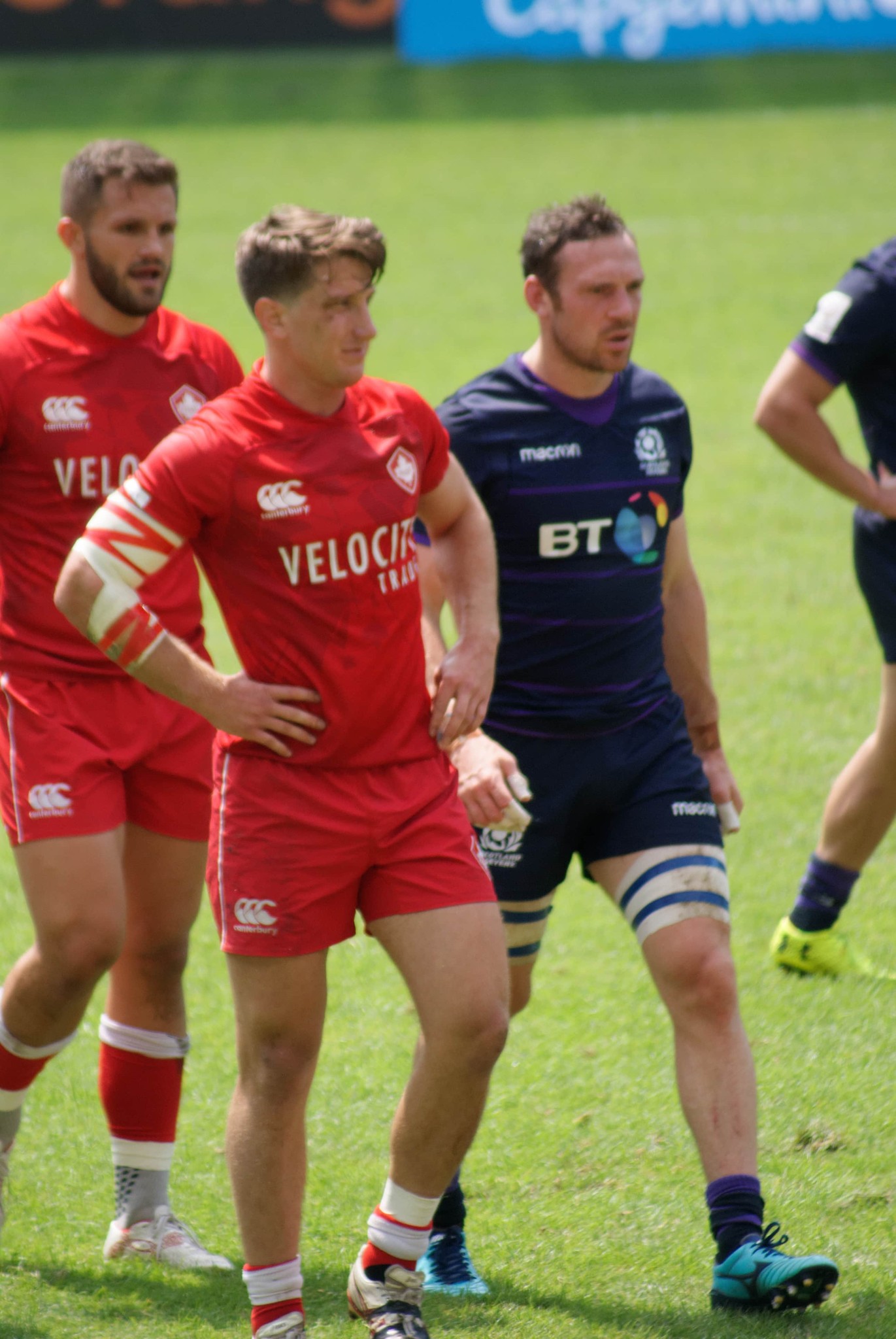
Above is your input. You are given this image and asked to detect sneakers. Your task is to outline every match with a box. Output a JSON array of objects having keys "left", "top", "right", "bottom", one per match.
[
  {"left": 347, "top": 1245, "right": 429, "bottom": 1339},
  {"left": 709, "top": 1222, "right": 839, "bottom": 1312},
  {"left": 769, "top": 917, "right": 896, "bottom": 982},
  {"left": 417, "top": 1188, "right": 489, "bottom": 1295},
  {"left": 104, "top": 1206, "right": 231, "bottom": 1269},
  {"left": 0, "top": 1142, "right": 13, "bottom": 1226}
]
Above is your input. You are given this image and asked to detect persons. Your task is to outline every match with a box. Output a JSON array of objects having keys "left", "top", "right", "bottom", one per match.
[
  {"left": 750, "top": 236, "right": 896, "bottom": 986},
  {"left": 411, "top": 192, "right": 837, "bottom": 1316},
  {"left": 0, "top": 138, "right": 246, "bottom": 1270},
  {"left": 53, "top": 204, "right": 501, "bottom": 1339}
]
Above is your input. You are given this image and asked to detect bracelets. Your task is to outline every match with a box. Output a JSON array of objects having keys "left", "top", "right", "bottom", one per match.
[{"left": 689, "top": 722, "right": 720, "bottom": 754}]
